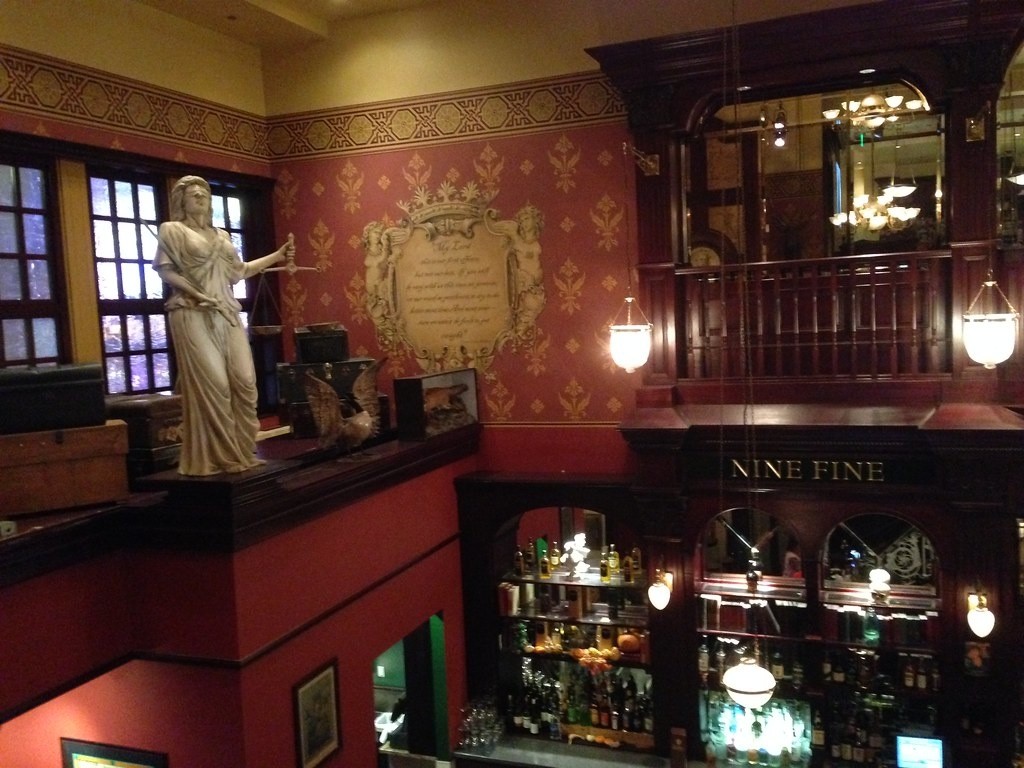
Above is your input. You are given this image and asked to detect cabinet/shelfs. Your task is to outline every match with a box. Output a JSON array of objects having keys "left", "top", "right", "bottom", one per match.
[
  {"left": 818, "top": 591, "right": 944, "bottom": 768},
  {"left": 696, "top": 581, "right": 809, "bottom": 763},
  {"left": 502, "top": 569, "right": 655, "bottom": 752}
]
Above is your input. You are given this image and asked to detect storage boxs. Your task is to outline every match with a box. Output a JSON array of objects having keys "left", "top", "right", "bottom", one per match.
[
  {"left": 277, "top": 357, "right": 377, "bottom": 403},
  {"left": 293, "top": 323, "right": 350, "bottom": 363},
  {"left": 248, "top": 326, "right": 284, "bottom": 420},
  {"left": 0, "top": 420, "right": 127, "bottom": 518},
  {"left": 278, "top": 391, "right": 391, "bottom": 441},
  {"left": 0, "top": 362, "right": 106, "bottom": 436}
]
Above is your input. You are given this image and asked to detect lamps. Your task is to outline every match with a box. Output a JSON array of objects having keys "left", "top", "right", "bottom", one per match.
[
  {"left": 821, "top": 88, "right": 921, "bottom": 233},
  {"left": 648, "top": 557, "right": 675, "bottom": 611},
  {"left": 759, "top": 106, "right": 789, "bottom": 150},
  {"left": 718, "top": 0, "right": 778, "bottom": 711},
  {"left": 869, "top": 569, "right": 891, "bottom": 606},
  {"left": 961, "top": 99, "right": 1020, "bottom": 370},
  {"left": 607, "top": 140, "right": 661, "bottom": 376},
  {"left": 967, "top": 574, "right": 995, "bottom": 638}
]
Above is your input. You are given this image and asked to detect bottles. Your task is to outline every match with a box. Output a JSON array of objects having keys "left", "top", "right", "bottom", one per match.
[
  {"left": 811, "top": 691, "right": 895, "bottom": 764},
  {"left": 508, "top": 680, "right": 654, "bottom": 740},
  {"left": 931, "top": 664, "right": 941, "bottom": 692},
  {"left": 905, "top": 654, "right": 914, "bottom": 689},
  {"left": 540, "top": 550, "right": 551, "bottom": 579},
  {"left": 550, "top": 542, "right": 560, "bottom": 571},
  {"left": 600, "top": 553, "right": 610, "bottom": 583},
  {"left": 526, "top": 537, "right": 535, "bottom": 571},
  {"left": 746, "top": 561, "right": 757, "bottom": 591},
  {"left": 712, "top": 703, "right": 804, "bottom": 768},
  {"left": 608, "top": 544, "right": 620, "bottom": 574},
  {"left": 823, "top": 659, "right": 845, "bottom": 683},
  {"left": 623, "top": 552, "right": 634, "bottom": 583},
  {"left": 514, "top": 545, "right": 524, "bottom": 577},
  {"left": 916, "top": 655, "right": 926, "bottom": 689},
  {"left": 632, "top": 544, "right": 642, "bottom": 574},
  {"left": 752, "top": 551, "right": 762, "bottom": 580},
  {"left": 699, "top": 635, "right": 803, "bottom": 690}
]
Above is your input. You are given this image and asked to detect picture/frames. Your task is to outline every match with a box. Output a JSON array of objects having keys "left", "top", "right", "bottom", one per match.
[
  {"left": 291, "top": 657, "right": 343, "bottom": 768},
  {"left": 59, "top": 737, "right": 170, "bottom": 768}
]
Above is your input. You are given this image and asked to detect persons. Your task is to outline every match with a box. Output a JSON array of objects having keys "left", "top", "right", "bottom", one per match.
[{"left": 153, "top": 172, "right": 296, "bottom": 478}]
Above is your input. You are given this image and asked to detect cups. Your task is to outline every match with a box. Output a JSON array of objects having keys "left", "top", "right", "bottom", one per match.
[
  {"left": 869, "top": 583, "right": 891, "bottom": 602},
  {"left": 869, "top": 569, "right": 890, "bottom": 586},
  {"left": 460, "top": 699, "right": 503, "bottom": 755}
]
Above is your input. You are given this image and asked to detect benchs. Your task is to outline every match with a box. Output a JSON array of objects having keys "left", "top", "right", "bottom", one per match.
[{"left": 105, "top": 393, "right": 185, "bottom": 476}]
